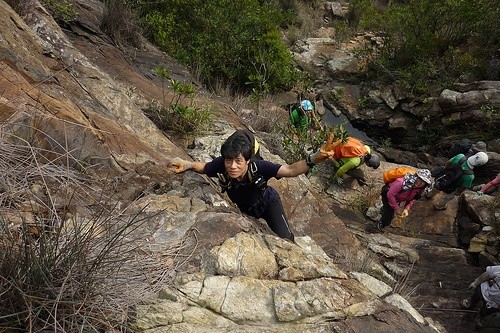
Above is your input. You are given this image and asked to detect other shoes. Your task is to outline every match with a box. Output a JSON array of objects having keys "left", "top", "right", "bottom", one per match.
[
  {"left": 435, "top": 204, "right": 447, "bottom": 210},
  {"left": 425, "top": 192, "right": 431, "bottom": 200},
  {"left": 377, "top": 221, "right": 385, "bottom": 232},
  {"left": 380, "top": 206, "right": 384, "bottom": 213}
]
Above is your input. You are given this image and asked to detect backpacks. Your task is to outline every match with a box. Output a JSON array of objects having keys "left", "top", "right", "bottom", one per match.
[
  {"left": 219, "top": 129, "right": 264, "bottom": 193},
  {"left": 434, "top": 156, "right": 476, "bottom": 194},
  {"left": 383, "top": 167, "right": 416, "bottom": 184},
  {"left": 333, "top": 137, "right": 367, "bottom": 160}
]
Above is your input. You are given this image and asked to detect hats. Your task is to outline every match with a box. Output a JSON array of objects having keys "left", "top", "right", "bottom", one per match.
[
  {"left": 369, "top": 154, "right": 380, "bottom": 169},
  {"left": 468, "top": 152, "right": 488, "bottom": 166},
  {"left": 417, "top": 169, "right": 432, "bottom": 185},
  {"left": 301, "top": 101, "right": 314, "bottom": 111}
]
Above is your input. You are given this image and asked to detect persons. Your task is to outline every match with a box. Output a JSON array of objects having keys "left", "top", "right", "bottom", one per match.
[
  {"left": 291, "top": 100, "right": 323, "bottom": 131},
  {"left": 318, "top": 145, "right": 380, "bottom": 185},
  {"left": 168, "top": 131, "right": 342, "bottom": 242},
  {"left": 378, "top": 169, "right": 431, "bottom": 232},
  {"left": 425, "top": 152, "right": 489, "bottom": 210},
  {"left": 461, "top": 265, "right": 500, "bottom": 328},
  {"left": 473, "top": 176, "right": 500, "bottom": 195}
]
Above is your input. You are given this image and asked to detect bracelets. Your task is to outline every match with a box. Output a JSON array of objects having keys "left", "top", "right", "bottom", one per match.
[{"left": 306, "top": 155, "right": 316, "bottom": 169}]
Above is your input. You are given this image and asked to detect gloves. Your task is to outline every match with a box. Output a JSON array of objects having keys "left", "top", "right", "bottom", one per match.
[
  {"left": 168, "top": 157, "right": 198, "bottom": 174},
  {"left": 310, "top": 134, "right": 342, "bottom": 165},
  {"left": 335, "top": 178, "right": 344, "bottom": 186},
  {"left": 401, "top": 209, "right": 408, "bottom": 218},
  {"left": 397, "top": 216, "right": 403, "bottom": 225},
  {"left": 468, "top": 281, "right": 479, "bottom": 291}
]
